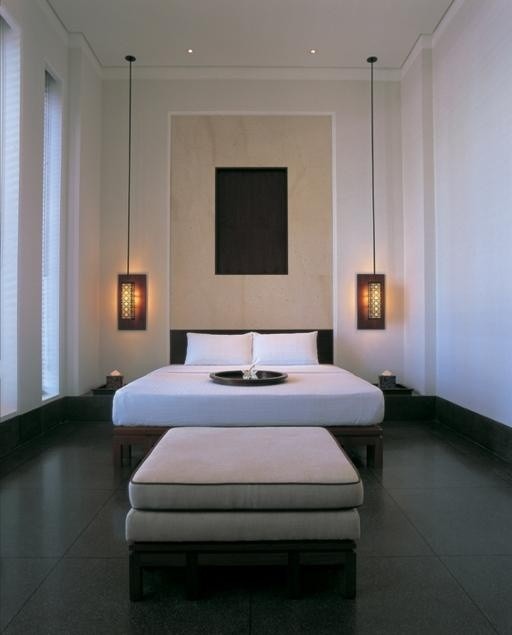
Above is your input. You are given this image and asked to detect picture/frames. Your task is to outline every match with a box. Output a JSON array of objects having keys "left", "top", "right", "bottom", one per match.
[
  {"left": 166, "top": 111, "right": 338, "bottom": 365},
  {"left": 354, "top": 272, "right": 388, "bottom": 333},
  {"left": 115, "top": 271, "right": 149, "bottom": 332}
]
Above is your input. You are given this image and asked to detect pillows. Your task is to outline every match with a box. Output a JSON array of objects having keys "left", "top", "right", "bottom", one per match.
[
  {"left": 184, "top": 332, "right": 253, "bottom": 366},
  {"left": 253, "top": 331, "right": 320, "bottom": 365}
]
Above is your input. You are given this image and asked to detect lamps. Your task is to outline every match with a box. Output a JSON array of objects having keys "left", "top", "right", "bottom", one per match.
[
  {"left": 368, "top": 57, "right": 381, "bottom": 319},
  {"left": 379, "top": 371, "right": 395, "bottom": 388},
  {"left": 121, "top": 55, "right": 135, "bottom": 319},
  {"left": 108, "top": 370, "right": 122, "bottom": 389}
]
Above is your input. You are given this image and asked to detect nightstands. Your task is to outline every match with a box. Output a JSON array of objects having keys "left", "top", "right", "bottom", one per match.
[
  {"left": 372, "top": 384, "right": 413, "bottom": 394},
  {"left": 92, "top": 385, "right": 126, "bottom": 395}
]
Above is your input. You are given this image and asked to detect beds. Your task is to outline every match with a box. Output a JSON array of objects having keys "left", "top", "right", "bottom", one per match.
[{"left": 112, "top": 329, "right": 385, "bottom": 469}]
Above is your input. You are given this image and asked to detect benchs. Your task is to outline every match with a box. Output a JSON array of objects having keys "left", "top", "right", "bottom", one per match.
[{"left": 126, "top": 425, "right": 364, "bottom": 601}]
